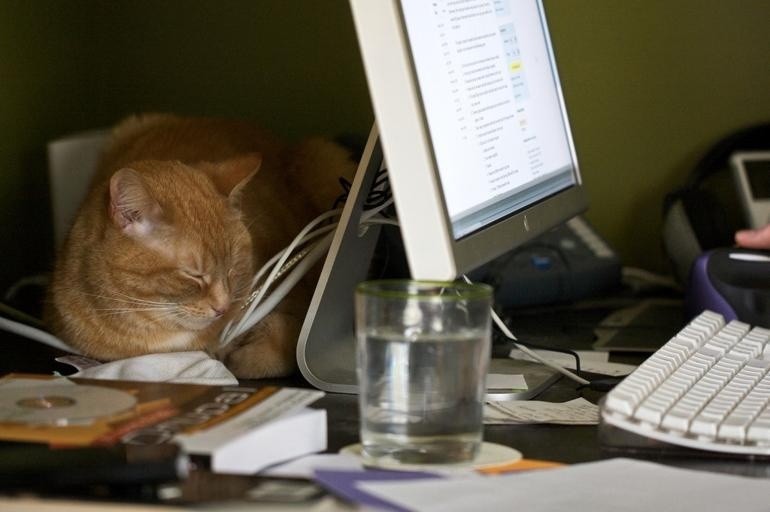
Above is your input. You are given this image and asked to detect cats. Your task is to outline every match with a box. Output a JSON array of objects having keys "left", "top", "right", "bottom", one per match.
[{"left": 39, "top": 107, "right": 359, "bottom": 383}]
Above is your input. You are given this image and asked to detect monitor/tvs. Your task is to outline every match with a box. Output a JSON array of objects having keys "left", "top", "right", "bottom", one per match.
[{"left": 296, "top": 0, "right": 587, "bottom": 405}]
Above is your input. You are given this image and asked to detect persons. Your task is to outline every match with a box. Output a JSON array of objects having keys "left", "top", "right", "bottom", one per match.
[{"left": 732, "top": 219, "right": 770, "bottom": 250}]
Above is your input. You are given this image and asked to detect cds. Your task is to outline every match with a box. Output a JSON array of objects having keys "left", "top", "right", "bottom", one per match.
[{"left": 0, "top": 376, "right": 137, "bottom": 429}]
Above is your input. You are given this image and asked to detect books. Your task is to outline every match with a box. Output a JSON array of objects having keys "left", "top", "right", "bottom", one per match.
[{"left": 0, "top": 376, "right": 327, "bottom": 481}]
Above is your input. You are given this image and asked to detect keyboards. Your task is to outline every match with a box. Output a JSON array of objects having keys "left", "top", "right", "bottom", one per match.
[{"left": 600, "top": 309, "right": 770, "bottom": 462}]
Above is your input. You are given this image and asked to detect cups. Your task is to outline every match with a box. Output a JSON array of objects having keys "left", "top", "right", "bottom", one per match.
[{"left": 351, "top": 277, "right": 495, "bottom": 467}]
